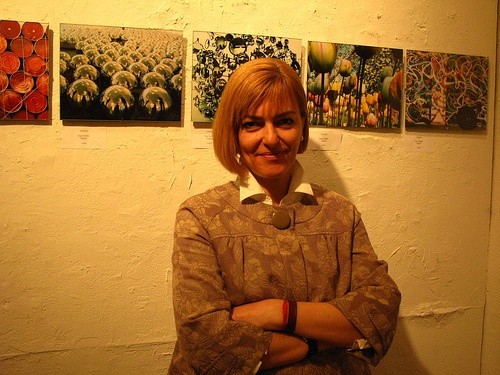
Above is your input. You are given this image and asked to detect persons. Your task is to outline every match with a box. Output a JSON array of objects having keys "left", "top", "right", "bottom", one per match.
[{"left": 166, "top": 58, "right": 401, "bottom": 375}]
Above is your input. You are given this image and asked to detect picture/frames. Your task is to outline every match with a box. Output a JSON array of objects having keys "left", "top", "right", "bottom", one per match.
[
  {"left": 60, "top": 23, "right": 182, "bottom": 122},
  {"left": 0, "top": 19, "right": 50, "bottom": 121},
  {"left": 191, "top": 30, "right": 302, "bottom": 122},
  {"left": 405, "top": 49, "right": 489, "bottom": 130},
  {"left": 306, "top": 40, "right": 403, "bottom": 128}
]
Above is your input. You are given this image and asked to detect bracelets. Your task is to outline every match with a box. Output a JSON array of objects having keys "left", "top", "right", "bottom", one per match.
[{"left": 282, "top": 298, "right": 297, "bottom": 333}]
